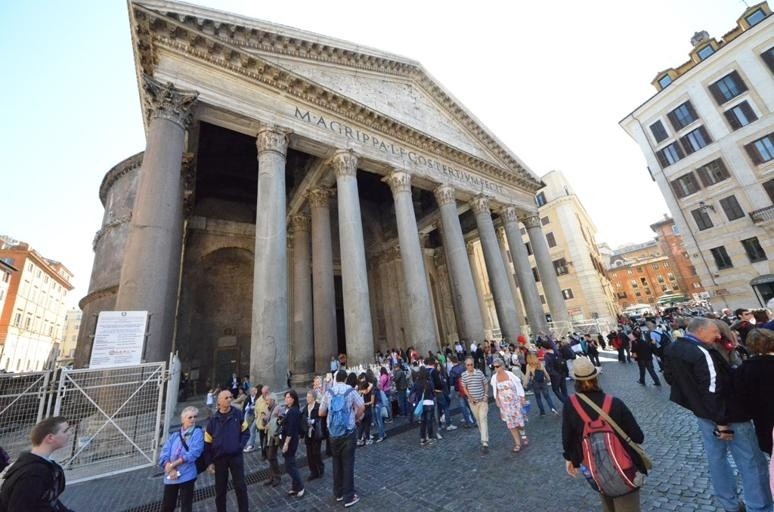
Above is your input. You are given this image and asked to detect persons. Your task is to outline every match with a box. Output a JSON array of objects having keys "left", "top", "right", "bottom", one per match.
[
  {"left": 560, "top": 358, "right": 645, "bottom": 511},
  {"left": 205, "top": 390, "right": 250, "bottom": 511},
  {"left": 661, "top": 316, "right": 774, "bottom": 511},
  {"left": 377, "top": 346, "right": 418, "bottom": 370},
  {"left": 491, "top": 358, "right": 528, "bottom": 452},
  {"left": 227, "top": 373, "right": 241, "bottom": 399},
  {"left": 460, "top": 358, "right": 490, "bottom": 453},
  {"left": 331, "top": 354, "right": 346, "bottom": 372},
  {"left": 523, "top": 353, "right": 559, "bottom": 417},
  {"left": 158, "top": 406, "right": 204, "bottom": 512},
  {"left": 319, "top": 370, "right": 365, "bottom": 507},
  {"left": 1, "top": 415, "right": 73, "bottom": 511},
  {"left": 731, "top": 327, "right": 774, "bottom": 456},
  {"left": 540, "top": 341, "right": 569, "bottom": 403},
  {"left": 445, "top": 331, "right": 600, "bottom": 395},
  {"left": 206, "top": 387, "right": 219, "bottom": 416},
  {"left": 242, "top": 375, "right": 250, "bottom": 392},
  {"left": 233, "top": 372, "right": 333, "bottom": 499},
  {"left": 598, "top": 333, "right": 606, "bottom": 349},
  {"left": 608, "top": 308, "right": 774, "bottom": 386},
  {"left": 346, "top": 351, "right": 478, "bottom": 446}
]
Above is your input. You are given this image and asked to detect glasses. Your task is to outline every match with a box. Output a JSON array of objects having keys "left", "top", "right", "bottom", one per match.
[{"left": 493, "top": 366, "right": 499, "bottom": 368}]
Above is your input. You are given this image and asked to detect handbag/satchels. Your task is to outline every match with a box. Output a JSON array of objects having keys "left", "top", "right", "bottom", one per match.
[
  {"left": 414, "top": 400, "right": 423, "bottom": 416},
  {"left": 314, "top": 418, "right": 327, "bottom": 441},
  {"left": 521, "top": 400, "right": 531, "bottom": 414},
  {"left": 396, "top": 378, "right": 407, "bottom": 390},
  {"left": 375, "top": 390, "right": 390, "bottom": 406},
  {"left": 449, "top": 391, "right": 465, "bottom": 412},
  {"left": 534, "top": 368, "right": 544, "bottom": 383},
  {"left": 195, "top": 452, "right": 210, "bottom": 473},
  {"left": 300, "top": 411, "right": 309, "bottom": 438},
  {"left": 629, "top": 439, "right": 651, "bottom": 475}
]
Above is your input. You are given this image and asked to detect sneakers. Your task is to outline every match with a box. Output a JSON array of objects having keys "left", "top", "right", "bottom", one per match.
[
  {"left": 652, "top": 382, "right": 661, "bottom": 385},
  {"left": 637, "top": 379, "right": 645, "bottom": 384},
  {"left": 513, "top": 437, "right": 528, "bottom": 452},
  {"left": 244, "top": 445, "right": 255, "bottom": 453},
  {"left": 336, "top": 493, "right": 360, "bottom": 507},
  {"left": 264, "top": 470, "right": 326, "bottom": 496},
  {"left": 418, "top": 424, "right": 458, "bottom": 445},
  {"left": 540, "top": 408, "right": 560, "bottom": 417},
  {"left": 356, "top": 433, "right": 387, "bottom": 446},
  {"left": 480, "top": 447, "right": 489, "bottom": 453},
  {"left": 460, "top": 416, "right": 477, "bottom": 428},
  {"left": 382, "top": 418, "right": 394, "bottom": 424}
]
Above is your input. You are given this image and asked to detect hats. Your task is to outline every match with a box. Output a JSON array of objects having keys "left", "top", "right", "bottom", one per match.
[{"left": 570, "top": 358, "right": 602, "bottom": 380}]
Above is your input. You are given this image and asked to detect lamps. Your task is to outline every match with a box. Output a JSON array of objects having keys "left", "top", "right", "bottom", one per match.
[{"left": 699, "top": 201, "right": 717, "bottom": 215}]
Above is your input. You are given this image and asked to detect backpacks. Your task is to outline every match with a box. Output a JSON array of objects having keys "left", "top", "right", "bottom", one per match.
[
  {"left": 651, "top": 330, "right": 671, "bottom": 347},
  {"left": 327, "top": 388, "right": 354, "bottom": 440},
  {"left": 571, "top": 394, "right": 646, "bottom": 497},
  {"left": 551, "top": 353, "right": 569, "bottom": 377}
]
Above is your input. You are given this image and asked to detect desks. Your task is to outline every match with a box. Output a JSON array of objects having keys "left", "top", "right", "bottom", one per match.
[{"left": 211, "top": 346, "right": 241, "bottom": 387}]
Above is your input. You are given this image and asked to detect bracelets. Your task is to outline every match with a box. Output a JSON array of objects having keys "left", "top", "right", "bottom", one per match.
[{"left": 484, "top": 394, "right": 488, "bottom": 397}]
